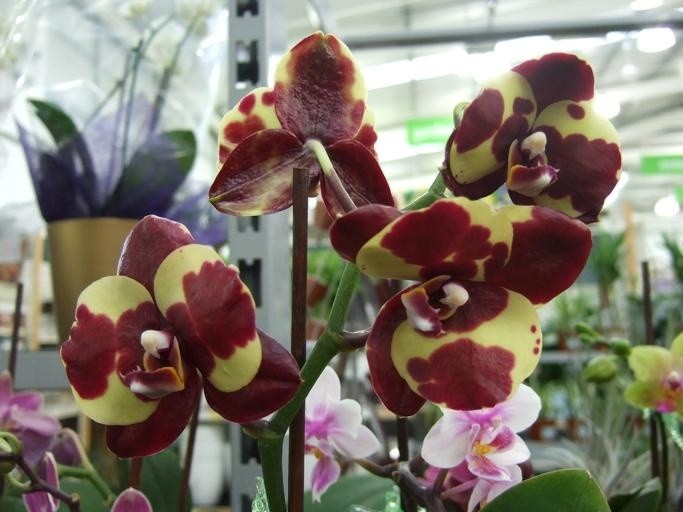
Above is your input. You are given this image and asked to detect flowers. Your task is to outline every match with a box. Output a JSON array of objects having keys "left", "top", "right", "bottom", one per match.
[{"left": 3, "top": 36, "right": 680, "bottom": 512}]
